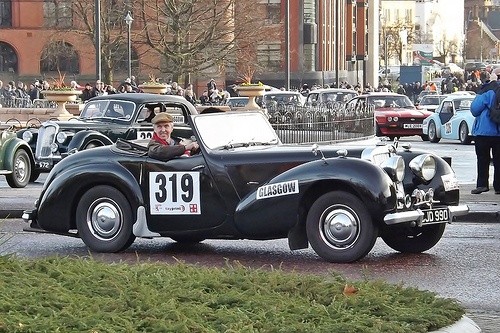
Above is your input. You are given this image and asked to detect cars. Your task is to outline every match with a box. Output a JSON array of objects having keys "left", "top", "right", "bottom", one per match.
[
  {"left": 222, "top": 89, "right": 306, "bottom": 128},
  {"left": 292, "top": 88, "right": 363, "bottom": 129},
  {"left": 22, "top": 110, "right": 471, "bottom": 264},
  {"left": 16, "top": 92, "right": 226, "bottom": 183},
  {"left": 422, "top": 95, "right": 476, "bottom": 145},
  {"left": 0, "top": 123, "right": 39, "bottom": 189},
  {"left": 417, "top": 77, "right": 477, "bottom": 113},
  {"left": 335, "top": 91, "right": 434, "bottom": 142}
]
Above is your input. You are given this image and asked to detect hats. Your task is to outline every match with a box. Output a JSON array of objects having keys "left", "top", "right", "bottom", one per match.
[{"left": 151, "top": 112, "right": 174, "bottom": 124}]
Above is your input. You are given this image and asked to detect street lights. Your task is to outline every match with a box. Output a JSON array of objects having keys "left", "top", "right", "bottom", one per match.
[{"left": 123, "top": 11, "right": 134, "bottom": 78}]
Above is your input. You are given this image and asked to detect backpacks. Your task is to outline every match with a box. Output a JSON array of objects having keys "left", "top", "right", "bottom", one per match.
[{"left": 483, "top": 87, "right": 500, "bottom": 124}]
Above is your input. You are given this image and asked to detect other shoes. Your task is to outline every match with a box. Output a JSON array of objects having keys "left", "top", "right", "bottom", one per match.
[
  {"left": 494, "top": 190, "right": 500, "bottom": 194},
  {"left": 471, "top": 186, "right": 489, "bottom": 194}
]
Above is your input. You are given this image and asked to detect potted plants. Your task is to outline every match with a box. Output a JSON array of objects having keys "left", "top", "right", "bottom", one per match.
[
  {"left": 40, "top": 67, "right": 83, "bottom": 116},
  {"left": 138, "top": 73, "right": 167, "bottom": 94},
  {"left": 237, "top": 66, "right": 264, "bottom": 110}
]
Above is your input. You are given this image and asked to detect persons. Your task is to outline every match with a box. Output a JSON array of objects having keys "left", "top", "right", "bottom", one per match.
[
  {"left": 165, "top": 78, "right": 241, "bottom": 104},
  {"left": 470, "top": 68, "right": 500, "bottom": 195},
  {"left": 0, "top": 79, "right": 52, "bottom": 102},
  {"left": 71, "top": 76, "right": 143, "bottom": 102},
  {"left": 441, "top": 64, "right": 498, "bottom": 94},
  {"left": 147, "top": 111, "right": 199, "bottom": 162},
  {"left": 281, "top": 80, "right": 438, "bottom": 106}
]
